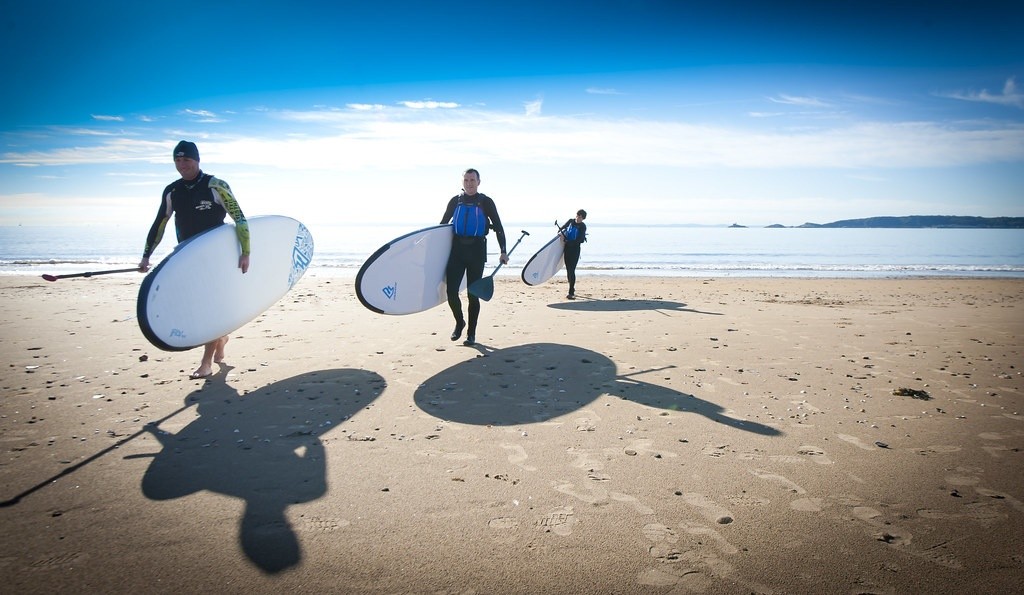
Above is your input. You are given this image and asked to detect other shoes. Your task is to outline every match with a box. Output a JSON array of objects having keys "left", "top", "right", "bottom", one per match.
[
  {"left": 450, "top": 320, "right": 466, "bottom": 342},
  {"left": 463, "top": 331, "right": 476, "bottom": 346},
  {"left": 567, "top": 292, "right": 576, "bottom": 298}
]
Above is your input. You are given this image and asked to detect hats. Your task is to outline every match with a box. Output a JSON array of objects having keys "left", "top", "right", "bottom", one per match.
[{"left": 173, "top": 140, "right": 201, "bottom": 161}]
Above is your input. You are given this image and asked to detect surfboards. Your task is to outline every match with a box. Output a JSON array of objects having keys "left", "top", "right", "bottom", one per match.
[
  {"left": 521, "top": 228, "right": 568, "bottom": 286},
  {"left": 354, "top": 223, "right": 485, "bottom": 316},
  {"left": 136, "top": 214, "right": 314, "bottom": 353}
]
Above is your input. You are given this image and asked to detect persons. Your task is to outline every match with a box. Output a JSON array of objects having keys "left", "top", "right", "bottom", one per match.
[
  {"left": 558, "top": 209, "right": 586, "bottom": 299},
  {"left": 139, "top": 141, "right": 250, "bottom": 377},
  {"left": 439, "top": 169, "right": 509, "bottom": 346}
]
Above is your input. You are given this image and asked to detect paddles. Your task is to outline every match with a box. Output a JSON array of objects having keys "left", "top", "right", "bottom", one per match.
[
  {"left": 554, "top": 220, "right": 568, "bottom": 240},
  {"left": 42, "top": 264, "right": 151, "bottom": 282},
  {"left": 468, "top": 230, "right": 530, "bottom": 301}
]
